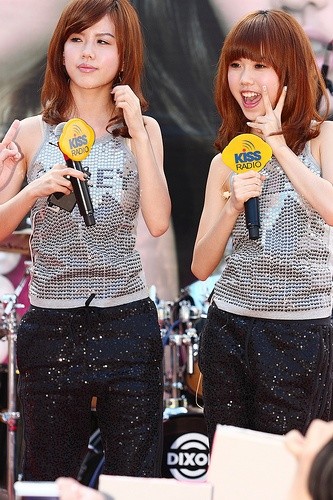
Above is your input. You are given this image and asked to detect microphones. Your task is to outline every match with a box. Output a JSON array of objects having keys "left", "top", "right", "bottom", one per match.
[
  {"left": 221, "top": 134, "right": 272, "bottom": 240},
  {"left": 53, "top": 118, "right": 95, "bottom": 227}
]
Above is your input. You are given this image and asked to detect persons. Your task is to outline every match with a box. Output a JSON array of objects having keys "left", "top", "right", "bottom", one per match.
[
  {"left": 0, "top": 0, "right": 171, "bottom": 500},
  {"left": 191, "top": 9, "right": 333, "bottom": 500}
]
